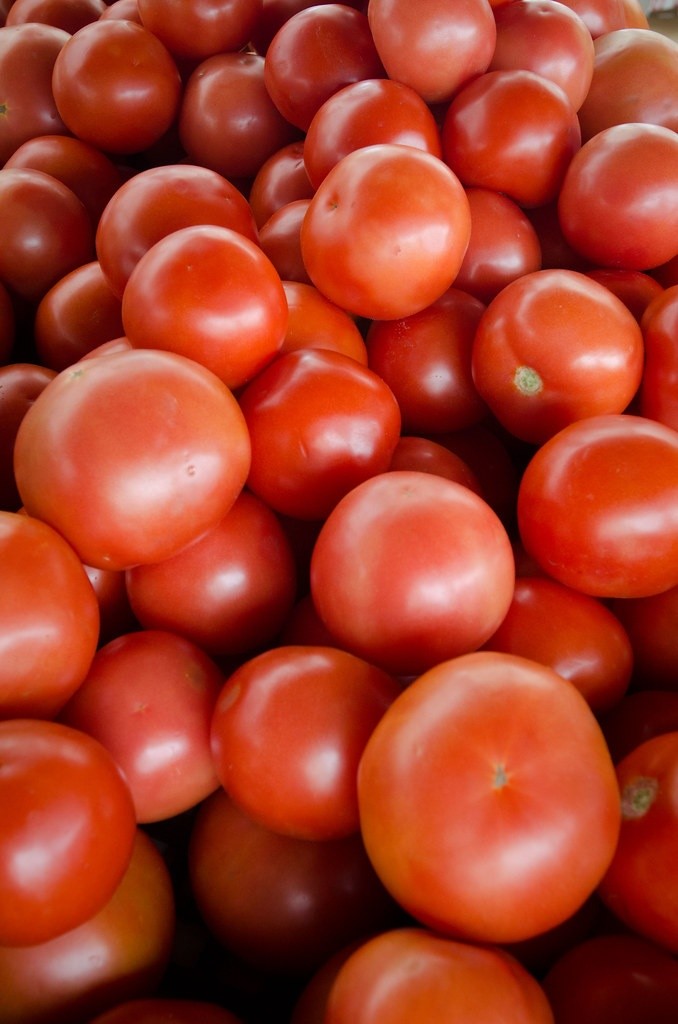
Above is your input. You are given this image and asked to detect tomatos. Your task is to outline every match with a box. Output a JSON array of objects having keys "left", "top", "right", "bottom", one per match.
[{"left": 0, "top": 0, "right": 678, "bottom": 1024}]
